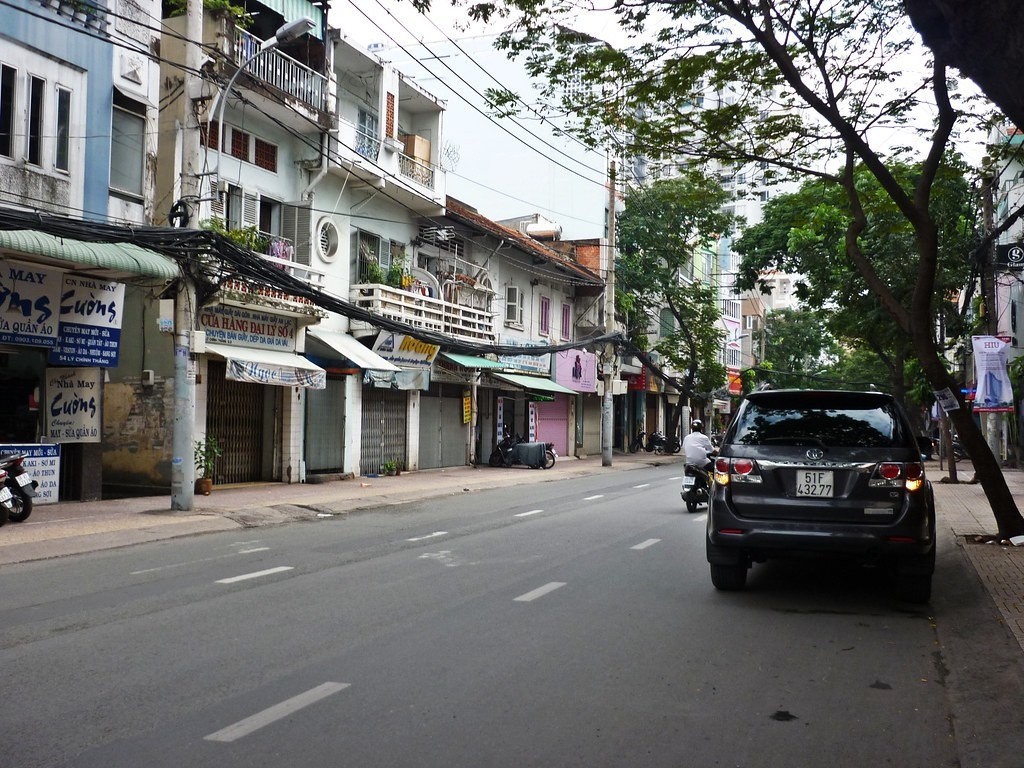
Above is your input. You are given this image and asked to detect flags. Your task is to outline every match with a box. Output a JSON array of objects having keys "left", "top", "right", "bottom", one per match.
[{"left": 971, "top": 335, "right": 1015, "bottom": 413}]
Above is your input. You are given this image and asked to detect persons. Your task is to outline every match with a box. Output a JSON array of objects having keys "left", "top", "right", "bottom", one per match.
[{"left": 683, "top": 419, "right": 720, "bottom": 473}]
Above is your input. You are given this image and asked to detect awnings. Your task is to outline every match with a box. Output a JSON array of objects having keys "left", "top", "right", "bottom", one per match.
[
  {"left": 438, "top": 350, "right": 507, "bottom": 369},
  {"left": 205, "top": 342, "right": 327, "bottom": 390},
  {"left": 307, "top": 326, "right": 402, "bottom": 374},
  {"left": 493, "top": 372, "right": 580, "bottom": 397}
]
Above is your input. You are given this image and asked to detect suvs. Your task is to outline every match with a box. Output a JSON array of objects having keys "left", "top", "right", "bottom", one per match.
[{"left": 704, "top": 385, "right": 935, "bottom": 593}]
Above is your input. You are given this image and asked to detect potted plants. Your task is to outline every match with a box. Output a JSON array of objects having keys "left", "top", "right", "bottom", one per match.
[
  {"left": 194, "top": 434, "right": 225, "bottom": 495},
  {"left": 385, "top": 459, "right": 401, "bottom": 476}
]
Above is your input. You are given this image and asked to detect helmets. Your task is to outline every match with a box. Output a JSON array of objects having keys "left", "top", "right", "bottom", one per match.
[{"left": 690, "top": 420, "right": 705, "bottom": 428}]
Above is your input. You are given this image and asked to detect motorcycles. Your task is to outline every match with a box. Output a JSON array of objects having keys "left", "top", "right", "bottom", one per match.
[
  {"left": 679, "top": 450, "right": 719, "bottom": 513},
  {"left": 489, "top": 423, "right": 559, "bottom": 470},
  {"left": 628, "top": 426, "right": 682, "bottom": 455},
  {"left": 0, "top": 451, "right": 40, "bottom": 527},
  {"left": 934, "top": 433, "right": 973, "bottom": 462}
]
